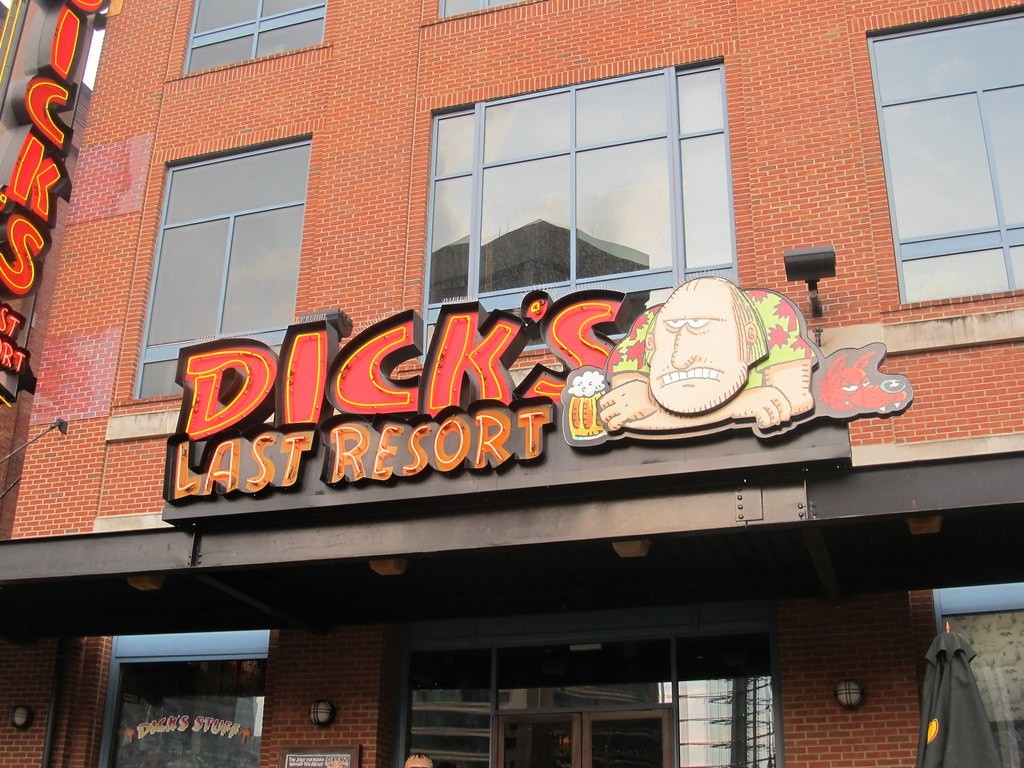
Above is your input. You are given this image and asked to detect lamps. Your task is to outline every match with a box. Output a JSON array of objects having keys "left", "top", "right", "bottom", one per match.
[
  {"left": 10, "top": 704, "right": 34, "bottom": 729},
  {"left": 370, "top": 558, "right": 410, "bottom": 577},
  {"left": 308, "top": 698, "right": 336, "bottom": 726},
  {"left": 781, "top": 245, "right": 837, "bottom": 318},
  {"left": 126, "top": 576, "right": 168, "bottom": 590},
  {"left": 833, "top": 679, "right": 865, "bottom": 709},
  {"left": 905, "top": 515, "right": 944, "bottom": 534},
  {"left": 611, "top": 539, "right": 653, "bottom": 558}
]
